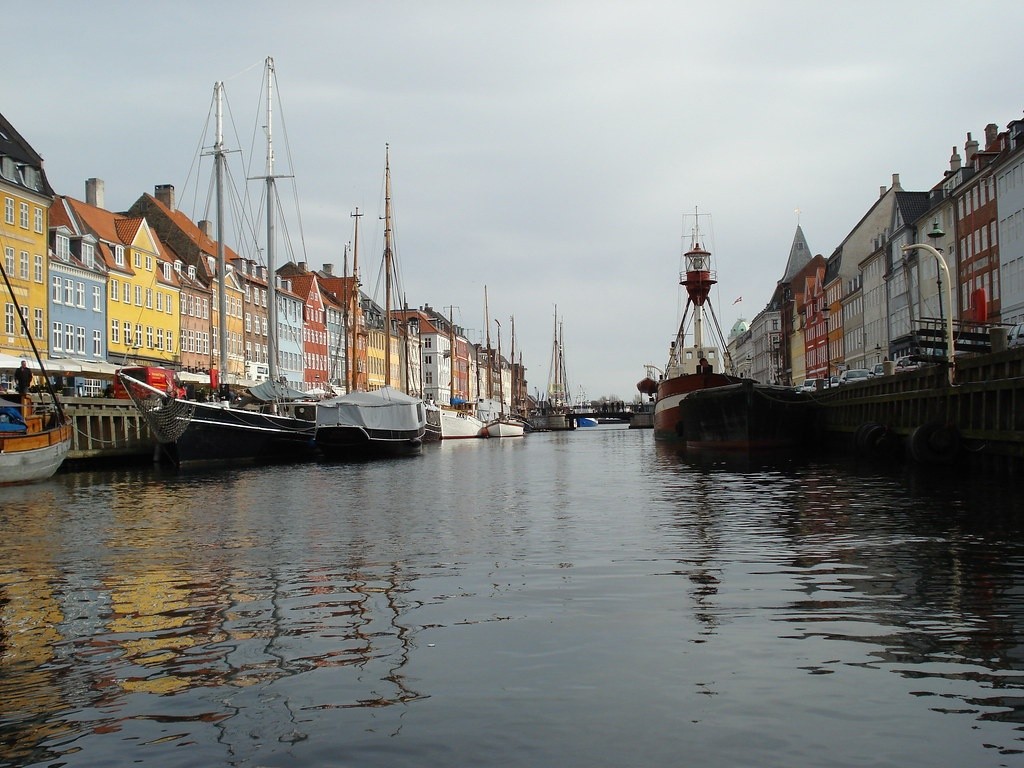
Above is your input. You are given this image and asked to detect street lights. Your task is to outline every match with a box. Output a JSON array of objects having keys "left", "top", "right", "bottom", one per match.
[
  {"left": 131, "top": 343, "right": 140, "bottom": 350},
  {"left": 874, "top": 341, "right": 883, "bottom": 363},
  {"left": 746, "top": 354, "right": 751, "bottom": 379},
  {"left": 926, "top": 220, "right": 946, "bottom": 242},
  {"left": 820, "top": 301, "right": 831, "bottom": 389},
  {"left": 773, "top": 340, "right": 781, "bottom": 384}
]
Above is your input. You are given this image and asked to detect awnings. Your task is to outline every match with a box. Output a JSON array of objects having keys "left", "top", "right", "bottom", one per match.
[{"left": 1, "top": 350, "right": 261, "bottom": 389}]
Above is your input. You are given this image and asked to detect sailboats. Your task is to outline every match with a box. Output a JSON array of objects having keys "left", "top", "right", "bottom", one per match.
[
  {"left": 115, "top": 55, "right": 314, "bottom": 470},
  {"left": 426, "top": 305, "right": 485, "bottom": 437},
  {"left": 654, "top": 205, "right": 743, "bottom": 438},
  {"left": 0, "top": 261, "right": 73, "bottom": 484},
  {"left": 529, "top": 306, "right": 569, "bottom": 429},
  {"left": 486, "top": 314, "right": 523, "bottom": 437},
  {"left": 315, "top": 143, "right": 427, "bottom": 455}
]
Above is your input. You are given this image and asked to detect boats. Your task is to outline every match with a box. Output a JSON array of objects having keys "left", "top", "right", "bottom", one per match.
[
  {"left": 679, "top": 383, "right": 800, "bottom": 474},
  {"left": 577, "top": 414, "right": 598, "bottom": 428},
  {"left": 630, "top": 392, "right": 654, "bottom": 428}
]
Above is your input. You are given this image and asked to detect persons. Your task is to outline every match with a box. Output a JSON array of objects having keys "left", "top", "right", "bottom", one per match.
[
  {"left": 601, "top": 401, "right": 625, "bottom": 412},
  {"left": 14, "top": 360, "right": 32, "bottom": 393}
]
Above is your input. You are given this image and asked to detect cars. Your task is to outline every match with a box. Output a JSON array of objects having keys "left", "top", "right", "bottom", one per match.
[{"left": 794, "top": 323, "right": 1023, "bottom": 394}]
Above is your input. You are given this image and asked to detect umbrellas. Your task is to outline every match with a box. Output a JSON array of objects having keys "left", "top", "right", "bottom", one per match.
[{"left": 303, "top": 388, "right": 333, "bottom": 396}]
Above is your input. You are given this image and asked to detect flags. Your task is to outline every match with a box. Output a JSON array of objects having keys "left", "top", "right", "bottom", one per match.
[{"left": 731, "top": 297, "right": 742, "bottom": 306}]
